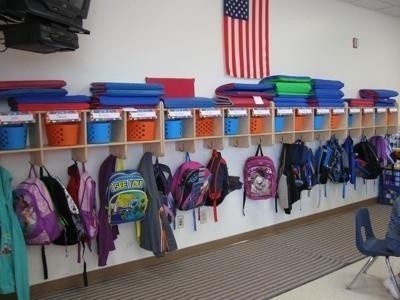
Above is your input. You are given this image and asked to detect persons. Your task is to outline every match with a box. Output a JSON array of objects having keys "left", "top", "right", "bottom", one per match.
[{"left": 376, "top": 189, "right": 400, "bottom": 300}]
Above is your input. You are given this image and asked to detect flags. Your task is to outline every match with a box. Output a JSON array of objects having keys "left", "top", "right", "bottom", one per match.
[{"left": 224, "top": 0, "right": 269, "bottom": 80}]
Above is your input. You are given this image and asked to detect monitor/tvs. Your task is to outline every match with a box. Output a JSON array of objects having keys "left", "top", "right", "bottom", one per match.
[{"left": 0, "top": 0, "right": 91, "bottom": 27}]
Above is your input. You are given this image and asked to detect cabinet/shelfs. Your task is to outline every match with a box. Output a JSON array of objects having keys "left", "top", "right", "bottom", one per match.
[{"left": 0, "top": 100, "right": 400, "bottom": 167}]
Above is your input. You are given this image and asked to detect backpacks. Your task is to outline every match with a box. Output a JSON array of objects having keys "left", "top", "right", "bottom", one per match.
[
  {"left": 243, "top": 144, "right": 278, "bottom": 216},
  {"left": 11, "top": 160, "right": 100, "bottom": 287},
  {"left": 103, "top": 157, "right": 149, "bottom": 245},
  {"left": 153, "top": 149, "right": 230, "bottom": 231},
  {"left": 276, "top": 135, "right": 395, "bottom": 214}
]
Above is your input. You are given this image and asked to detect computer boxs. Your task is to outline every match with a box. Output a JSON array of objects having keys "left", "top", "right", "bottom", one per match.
[{"left": 4, "top": 24, "right": 79, "bottom": 54}]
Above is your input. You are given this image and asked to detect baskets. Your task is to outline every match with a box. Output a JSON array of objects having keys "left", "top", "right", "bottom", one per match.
[
  {"left": 250, "top": 116, "right": 264, "bottom": 134},
  {"left": 0, "top": 124, "right": 27, "bottom": 150},
  {"left": 87, "top": 121, "right": 112, "bottom": 144},
  {"left": 224, "top": 117, "right": 239, "bottom": 135},
  {"left": 314, "top": 111, "right": 391, "bottom": 130},
  {"left": 127, "top": 119, "right": 155, "bottom": 141},
  {"left": 295, "top": 115, "right": 305, "bottom": 131},
  {"left": 275, "top": 115, "right": 285, "bottom": 132},
  {"left": 46, "top": 122, "right": 80, "bottom": 146},
  {"left": 164, "top": 118, "right": 183, "bottom": 139},
  {"left": 196, "top": 117, "right": 213, "bottom": 136}
]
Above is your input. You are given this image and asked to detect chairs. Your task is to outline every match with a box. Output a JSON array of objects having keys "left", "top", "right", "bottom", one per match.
[{"left": 347, "top": 207, "right": 400, "bottom": 298}]
